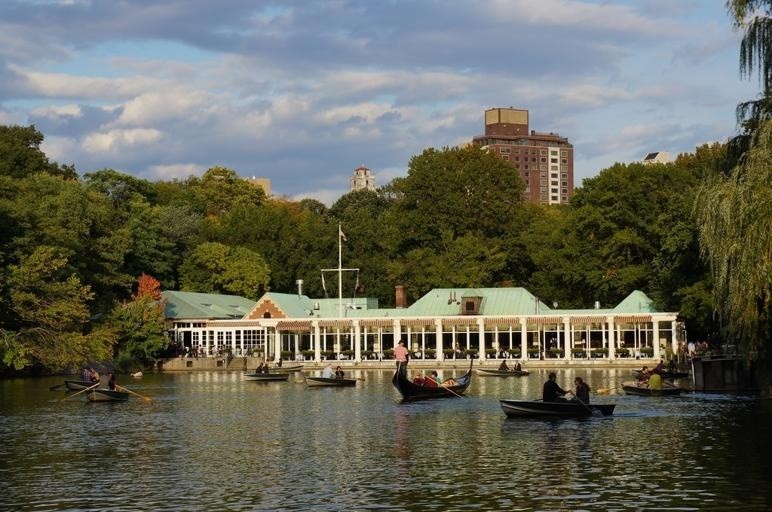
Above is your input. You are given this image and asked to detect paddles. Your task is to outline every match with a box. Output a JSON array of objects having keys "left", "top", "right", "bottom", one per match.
[
  {"left": 114, "top": 384, "right": 151, "bottom": 401},
  {"left": 569, "top": 390, "right": 604, "bottom": 418},
  {"left": 50, "top": 383, "right": 66, "bottom": 390},
  {"left": 597, "top": 385, "right": 624, "bottom": 396},
  {"left": 60, "top": 382, "right": 100, "bottom": 402}
]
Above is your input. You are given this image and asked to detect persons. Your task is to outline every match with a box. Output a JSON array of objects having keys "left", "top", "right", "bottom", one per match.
[
  {"left": 256, "top": 363, "right": 263, "bottom": 374},
  {"left": 432, "top": 371, "right": 442, "bottom": 385},
  {"left": 679, "top": 339, "right": 709, "bottom": 356},
  {"left": 393, "top": 338, "right": 409, "bottom": 378},
  {"left": 336, "top": 366, "right": 345, "bottom": 380},
  {"left": 570, "top": 376, "right": 592, "bottom": 405},
  {"left": 82, "top": 367, "right": 116, "bottom": 393},
  {"left": 638, "top": 359, "right": 676, "bottom": 389},
  {"left": 322, "top": 365, "right": 337, "bottom": 380},
  {"left": 499, "top": 360, "right": 509, "bottom": 371},
  {"left": 543, "top": 372, "right": 574, "bottom": 402},
  {"left": 263, "top": 364, "right": 270, "bottom": 375},
  {"left": 513, "top": 360, "right": 522, "bottom": 371}
]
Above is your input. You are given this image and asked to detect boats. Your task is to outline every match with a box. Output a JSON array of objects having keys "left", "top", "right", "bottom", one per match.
[
  {"left": 65, "top": 380, "right": 128, "bottom": 402},
  {"left": 243, "top": 365, "right": 304, "bottom": 380},
  {"left": 500, "top": 399, "right": 617, "bottom": 417},
  {"left": 306, "top": 377, "right": 356, "bottom": 387},
  {"left": 392, "top": 357, "right": 528, "bottom": 399},
  {"left": 622, "top": 369, "right": 688, "bottom": 395}
]
